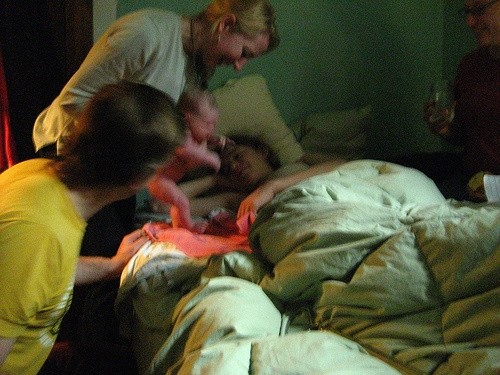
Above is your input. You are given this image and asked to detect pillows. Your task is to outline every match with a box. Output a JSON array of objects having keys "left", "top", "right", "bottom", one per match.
[
  {"left": 304, "top": 105, "right": 373, "bottom": 159},
  {"left": 211, "top": 73, "right": 304, "bottom": 164}
]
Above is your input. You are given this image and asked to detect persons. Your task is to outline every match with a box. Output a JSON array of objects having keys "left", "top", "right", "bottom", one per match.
[
  {"left": 32, "top": 0, "right": 281, "bottom": 356},
  {"left": 176, "top": 131, "right": 346, "bottom": 225},
  {"left": 0, "top": 79, "right": 182, "bottom": 375},
  {"left": 422, "top": 0, "right": 500, "bottom": 204},
  {"left": 144, "top": 87, "right": 226, "bottom": 234}
]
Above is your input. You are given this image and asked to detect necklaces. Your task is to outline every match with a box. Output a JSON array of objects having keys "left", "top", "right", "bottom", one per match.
[{"left": 189, "top": 14, "right": 210, "bottom": 90}]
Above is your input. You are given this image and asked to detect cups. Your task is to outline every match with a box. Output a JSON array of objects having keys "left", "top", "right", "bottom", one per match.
[{"left": 429, "top": 78, "right": 452, "bottom": 116}]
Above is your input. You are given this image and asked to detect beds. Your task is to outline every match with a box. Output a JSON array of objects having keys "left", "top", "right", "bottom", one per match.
[{"left": 110, "top": 149, "right": 500, "bottom": 375}]
